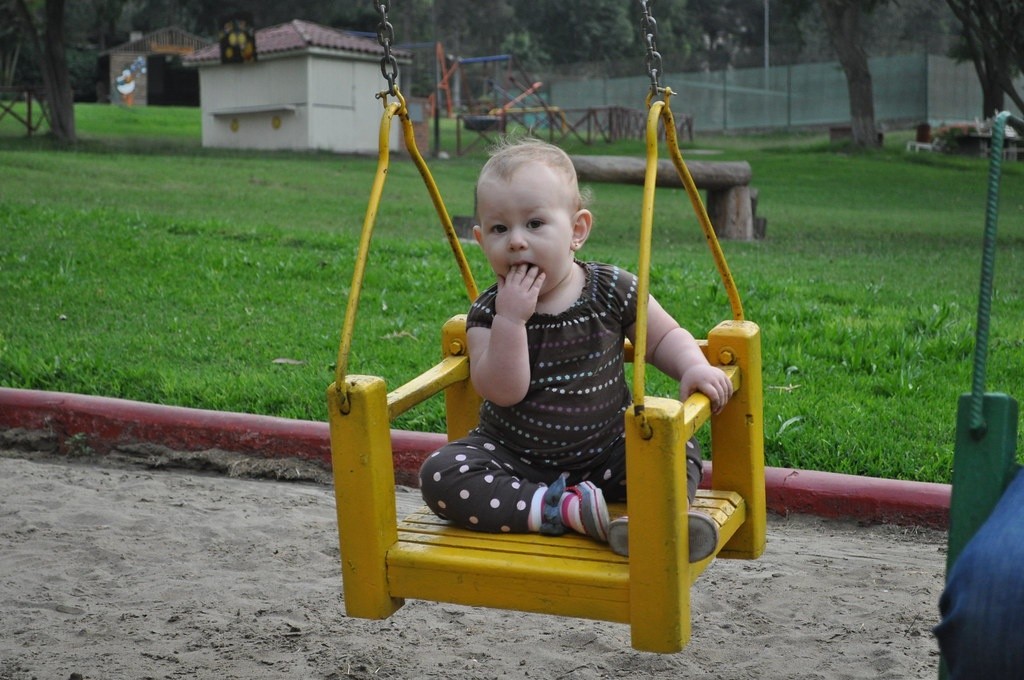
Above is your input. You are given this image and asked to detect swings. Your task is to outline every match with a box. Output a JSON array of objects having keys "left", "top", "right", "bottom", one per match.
[{"left": 324, "top": 0, "right": 769, "bottom": 655}]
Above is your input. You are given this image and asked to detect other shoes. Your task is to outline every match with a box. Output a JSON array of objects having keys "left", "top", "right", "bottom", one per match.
[
  {"left": 607, "top": 511, "right": 719, "bottom": 563},
  {"left": 567, "top": 479, "right": 610, "bottom": 542}
]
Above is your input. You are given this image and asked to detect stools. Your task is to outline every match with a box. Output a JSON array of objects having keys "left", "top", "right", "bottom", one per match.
[{"left": 907, "top": 140, "right": 932, "bottom": 154}]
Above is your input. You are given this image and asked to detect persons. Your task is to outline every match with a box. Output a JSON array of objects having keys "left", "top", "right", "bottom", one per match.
[
  {"left": 417, "top": 139, "right": 734, "bottom": 562},
  {"left": 932, "top": 467, "right": 1024, "bottom": 680}
]
